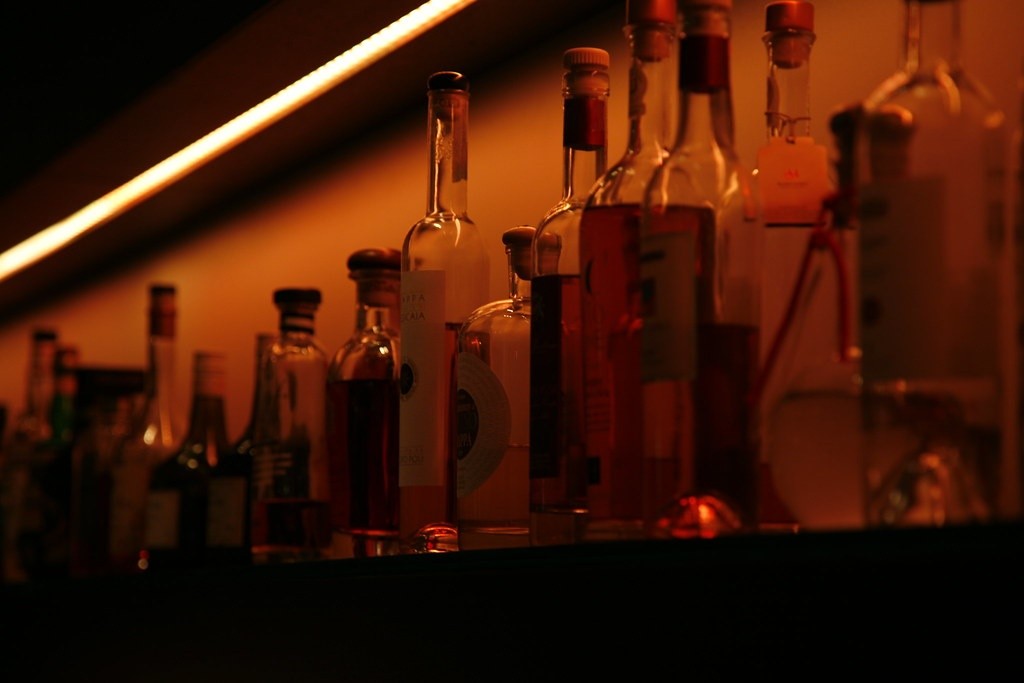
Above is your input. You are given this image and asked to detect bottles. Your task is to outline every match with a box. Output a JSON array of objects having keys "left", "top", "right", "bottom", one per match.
[
  {"left": 325, "top": 248, "right": 410, "bottom": 537},
  {"left": 17, "top": 349, "right": 80, "bottom": 573},
  {"left": 5, "top": 330, "right": 57, "bottom": 580},
  {"left": 580, "top": 0, "right": 680, "bottom": 531},
  {"left": 148, "top": 353, "right": 227, "bottom": 554},
  {"left": 530, "top": 47, "right": 611, "bottom": 532},
  {"left": 739, "top": 1, "right": 835, "bottom": 529},
  {"left": 262, "top": 289, "right": 328, "bottom": 552},
  {"left": 862, "top": 0, "right": 1014, "bottom": 531},
  {"left": 448, "top": 225, "right": 543, "bottom": 542},
  {"left": 400, "top": 71, "right": 490, "bottom": 544},
  {"left": 641, "top": 0, "right": 753, "bottom": 536},
  {"left": 111, "top": 285, "right": 188, "bottom": 563},
  {"left": 214, "top": 333, "right": 283, "bottom": 557}
]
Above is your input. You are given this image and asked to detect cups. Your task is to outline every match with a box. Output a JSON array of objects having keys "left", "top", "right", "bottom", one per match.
[{"left": 760, "top": 105, "right": 942, "bottom": 530}]
